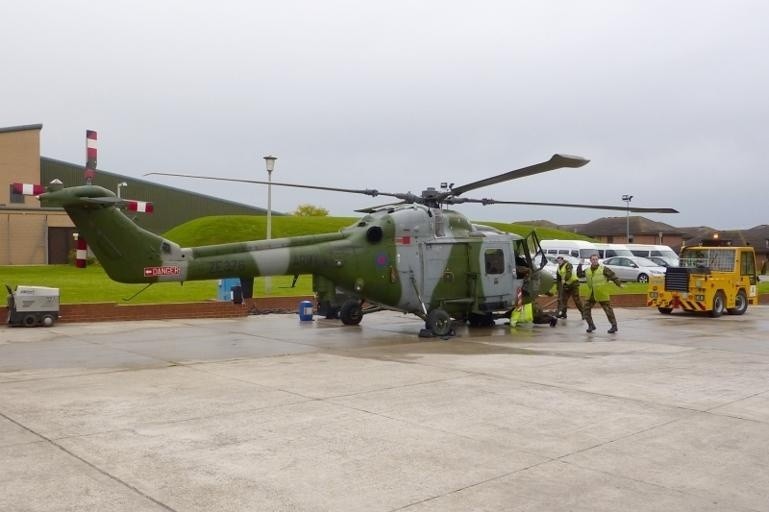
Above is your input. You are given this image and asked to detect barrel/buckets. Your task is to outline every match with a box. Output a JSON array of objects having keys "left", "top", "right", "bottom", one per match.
[
  {"left": 230, "top": 286, "right": 243, "bottom": 303},
  {"left": 299, "top": 301, "right": 313, "bottom": 321}
]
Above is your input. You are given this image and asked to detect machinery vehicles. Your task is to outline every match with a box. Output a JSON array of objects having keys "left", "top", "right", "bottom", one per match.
[{"left": 646, "top": 244, "right": 758, "bottom": 319}]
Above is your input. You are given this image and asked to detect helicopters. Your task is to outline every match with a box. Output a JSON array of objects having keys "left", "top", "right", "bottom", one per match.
[{"left": 9, "top": 126, "right": 678, "bottom": 338}]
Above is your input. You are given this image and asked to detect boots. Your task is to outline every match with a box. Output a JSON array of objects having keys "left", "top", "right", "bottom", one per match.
[
  {"left": 608, "top": 325, "right": 617, "bottom": 333},
  {"left": 587, "top": 324, "right": 595, "bottom": 332},
  {"left": 557, "top": 312, "right": 567, "bottom": 318}
]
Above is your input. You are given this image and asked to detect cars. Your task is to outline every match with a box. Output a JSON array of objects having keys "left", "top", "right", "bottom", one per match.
[
  {"left": 521, "top": 254, "right": 558, "bottom": 279},
  {"left": 540, "top": 254, "right": 589, "bottom": 281},
  {"left": 647, "top": 255, "right": 681, "bottom": 272},
  {"left": 598, "top": 255, "right": 667, "bottom": 284}
]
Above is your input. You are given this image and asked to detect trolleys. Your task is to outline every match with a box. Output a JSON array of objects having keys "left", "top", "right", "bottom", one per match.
[{"left": 2, "top": 281, "right": 60, "bottom": 328}]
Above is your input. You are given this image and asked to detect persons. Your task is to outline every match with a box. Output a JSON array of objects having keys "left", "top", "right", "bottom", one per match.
[
  {"left": 504, "top": 303, "right": 558, "bottom": 328},
  {"left": 556, "top": 257, "right": 586, "bottom": 320},
  {"left": 577, "top": 254, "right": 625, "bottom": 333}
]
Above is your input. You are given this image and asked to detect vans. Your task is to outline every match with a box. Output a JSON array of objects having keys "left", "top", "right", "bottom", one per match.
[
  {"left": 535, "top": 240, "right": 602, "bottom": 265},
  {"left": 592, "top": 244, "right": 677, "bottom": 269}
]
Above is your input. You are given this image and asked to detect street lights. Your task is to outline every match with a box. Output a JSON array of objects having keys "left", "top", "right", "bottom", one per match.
[{"left": 264, "top": 154, "right": 277, "bottom": 289}]
